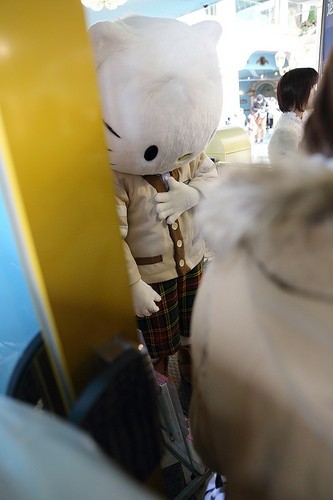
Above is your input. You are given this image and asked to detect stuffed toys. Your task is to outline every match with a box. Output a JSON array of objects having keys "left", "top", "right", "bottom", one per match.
[{"left": 86, "top": 14, "right": 223, "bottom": 418}]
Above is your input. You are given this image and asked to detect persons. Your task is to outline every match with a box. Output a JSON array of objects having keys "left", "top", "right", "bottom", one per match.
[
  {"left": 267, "top": 67, "right": 320, "bottom": 164},
  {"left": 252, "top": 94, "right": 279, "bottom": 143},
  {"left": 191, "top": 47, "right": 333, "bottom": 500}
]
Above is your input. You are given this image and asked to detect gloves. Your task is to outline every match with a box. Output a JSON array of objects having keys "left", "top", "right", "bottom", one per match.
[
  {"left": 154, "top": 177, "right": 199, "bottom": 224},
  {"left": 130, "top": 279, "right": 161, "bottom": 317}
]
